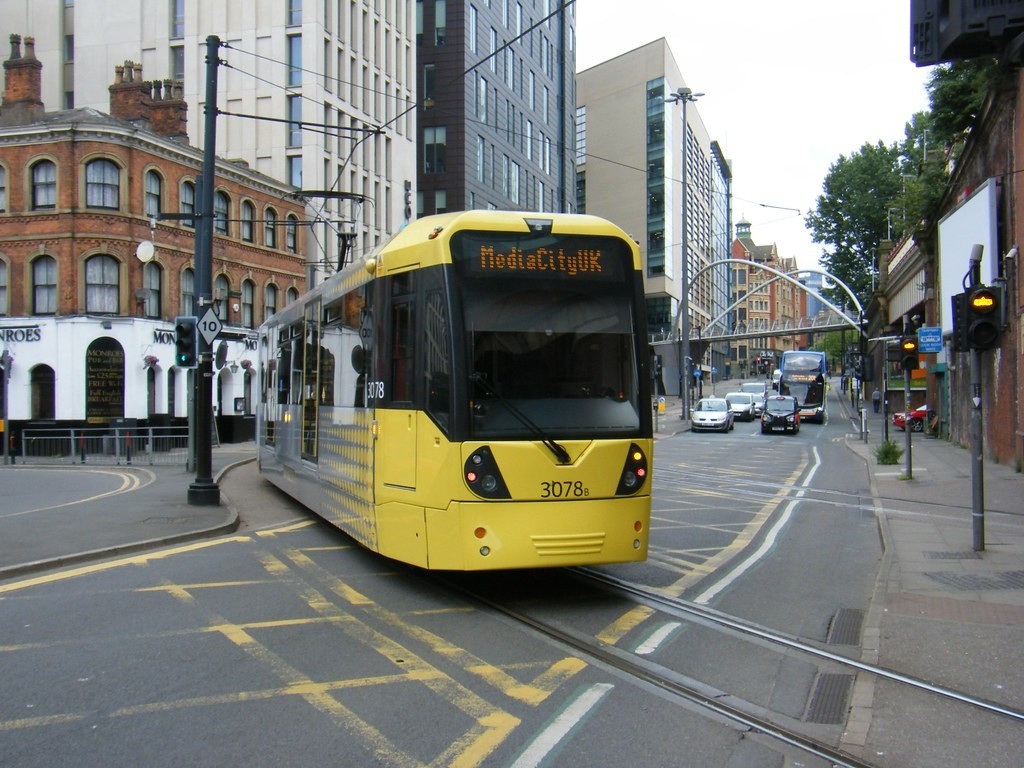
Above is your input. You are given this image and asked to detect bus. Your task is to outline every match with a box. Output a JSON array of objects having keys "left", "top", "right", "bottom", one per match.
[
  {"left": 250, "top": 211, "right": 653, "bottom": 571},
  {"left": 773, "top": 351, "right": 831, "bottom": 424}
]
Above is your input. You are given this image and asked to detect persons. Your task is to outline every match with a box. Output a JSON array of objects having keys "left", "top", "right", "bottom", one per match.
[{"left": 872, "top": 387, "right": 881, "bottom": 413}]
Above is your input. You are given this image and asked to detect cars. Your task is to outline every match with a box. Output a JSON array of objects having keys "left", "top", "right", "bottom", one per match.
[
  {"left": 892, "top": 405, "right": 926, "bottom": 433},
  {"left": 723, "top": 392, "right": 756, "bottom": 420},
  {"left": 741, "top": 383, "right": 768, "bottom": 416},
  {"left": 761, "top": 395, "right": 803, "bottom": 434},
  {"left": 772, "top": 369, "right": 782, "bottom": 389},
  {"left": 688, "top": 395, "right": 735, "bottom": 432}
]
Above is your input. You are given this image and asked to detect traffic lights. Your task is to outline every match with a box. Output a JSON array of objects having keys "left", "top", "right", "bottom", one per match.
[
  {"left": 174, "top": 316, "right": 199, "bottom": 370},
  {"left": 854, "top": 361, "right": 864, "bottom": 381},
  {"left": 901, "top": 337, "right": 920, "bottom": 370},
  {"left": 966, "top": 285, "right": 1003, "bottom": 352}
]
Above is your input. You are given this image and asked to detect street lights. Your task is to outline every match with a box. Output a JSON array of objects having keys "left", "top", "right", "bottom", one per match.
[{"left": 663, "top": 87, "right": 706, "bottom": 421}]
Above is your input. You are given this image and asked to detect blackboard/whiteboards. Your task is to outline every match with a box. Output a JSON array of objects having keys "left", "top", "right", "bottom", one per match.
[{"left": 210, "top": 406, "right": 219, "bottom": 446}]
